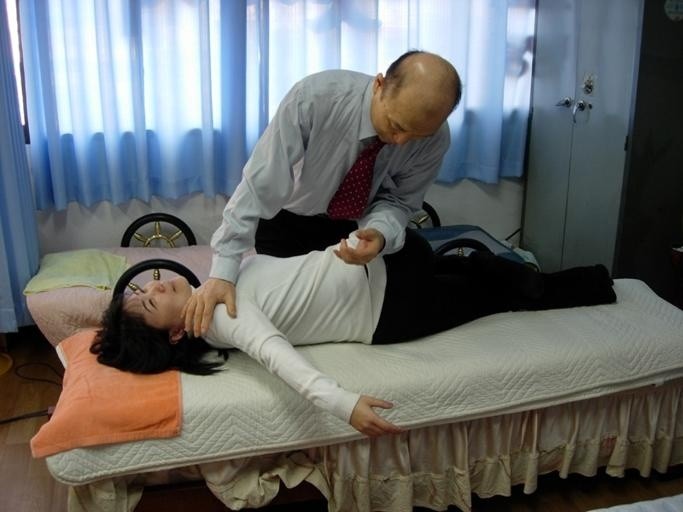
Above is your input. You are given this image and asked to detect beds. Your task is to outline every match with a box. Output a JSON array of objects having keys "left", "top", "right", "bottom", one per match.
[
  {"left": 24, "top": 201, "right": 541, "bottom": 350},
  {"left": 30, "top": 239, "right": 683, "bottom": 512}
]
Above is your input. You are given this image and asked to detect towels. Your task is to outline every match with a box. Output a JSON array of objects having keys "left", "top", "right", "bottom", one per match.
[
  {"left": 29, "top": 328, "right": 183, "bottom": 459},
  {"left": 22, "top": 249, "right": 130, "bottom": 295}
]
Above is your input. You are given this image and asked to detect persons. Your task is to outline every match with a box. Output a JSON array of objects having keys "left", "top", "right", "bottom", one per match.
[
  {"left": 180, "top": 50, "right": 461, "bottom": 340},
  {"left": 89, "top": 227, "right": 616, "bottom": 441}
]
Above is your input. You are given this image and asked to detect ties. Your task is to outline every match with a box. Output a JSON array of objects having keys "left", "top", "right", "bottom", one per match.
[{"left": 327, "top": 137, "right": 386, "bottom": 219}]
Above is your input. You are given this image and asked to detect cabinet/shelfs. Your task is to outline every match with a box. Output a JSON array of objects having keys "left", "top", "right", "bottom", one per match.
[{"left": 519, "top": 0, "right": 683, "bottom": 278}]
